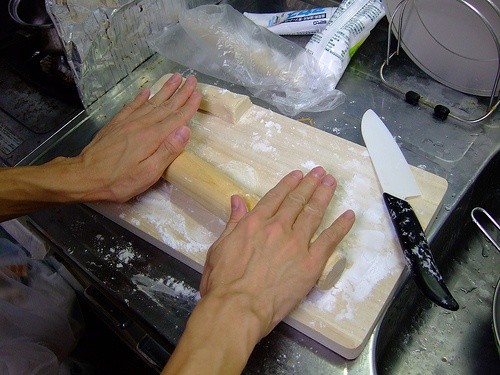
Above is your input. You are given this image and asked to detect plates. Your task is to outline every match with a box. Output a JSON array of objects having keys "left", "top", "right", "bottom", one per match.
[{"left": 382, "top": 0, "right": 500, "bottom": 97}]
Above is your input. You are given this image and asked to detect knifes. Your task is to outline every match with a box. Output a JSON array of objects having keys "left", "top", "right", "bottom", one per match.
[{"left": 360, "top": 108, "right": 460, "bottom": 311}]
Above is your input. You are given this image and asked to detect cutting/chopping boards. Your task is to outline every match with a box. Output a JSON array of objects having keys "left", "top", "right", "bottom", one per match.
[{"left": 83, "top": 73, "right": 449, "bottom": 359}]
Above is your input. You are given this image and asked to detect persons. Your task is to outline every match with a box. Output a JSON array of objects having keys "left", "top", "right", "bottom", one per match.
[{"left": 0, "top": 70, "right": 357, "bottom": 375}]
